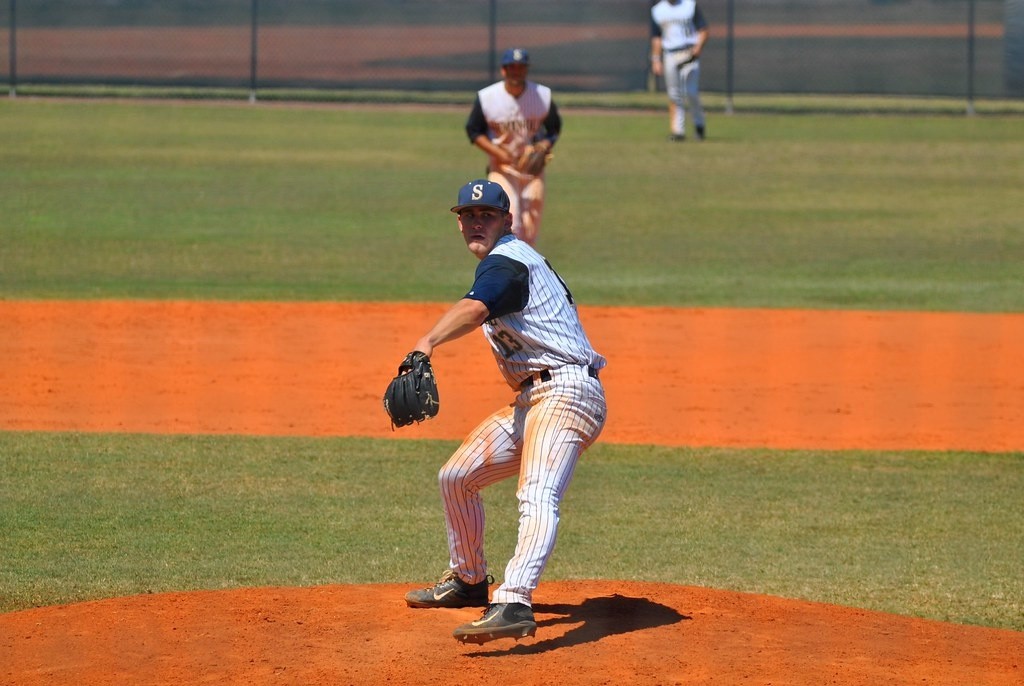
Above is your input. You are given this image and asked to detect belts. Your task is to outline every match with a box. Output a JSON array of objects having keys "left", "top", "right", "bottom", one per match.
[
  {"left": 668, "top": 44, "right": 693, "bottom": 52},
  {"left": 520, "top": 365, "right": 598, "bottom": 387}
]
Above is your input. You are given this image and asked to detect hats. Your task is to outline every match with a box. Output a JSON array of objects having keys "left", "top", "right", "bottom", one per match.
[
  {"left": 451, "top": 179, "right": 510, "bottom": 215},
  {"left": 501, "top": 48, "right": 528, "bottom": 67}
]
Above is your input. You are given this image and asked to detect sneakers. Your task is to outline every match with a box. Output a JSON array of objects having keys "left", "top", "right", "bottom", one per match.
[
  {"left": 453, "top": 600, "right": 537, "bottom": 646},
  {"left": 404, "top": 569, "right": 495, "bottom": 608}
]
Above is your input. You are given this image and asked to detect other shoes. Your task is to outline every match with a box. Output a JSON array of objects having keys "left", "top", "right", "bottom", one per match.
[
  {"left": 695, "top": 126, "right": 705, "bottom": 139},
  {"left": 667, "top": 134, "right": 684, "bottom": 142}
]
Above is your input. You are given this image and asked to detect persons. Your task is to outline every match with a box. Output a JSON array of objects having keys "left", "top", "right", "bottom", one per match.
[
  {"left": 466, "top": 47, "right": 562, "bottom": 243},
  {"left": 649, "top": 0, "right": 709, "bottom": 142},
  {"left": 385, "top": 178, "right": 607, "bottom": 646}
]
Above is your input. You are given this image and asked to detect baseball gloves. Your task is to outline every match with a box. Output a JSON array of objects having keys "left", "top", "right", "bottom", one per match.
[
  {"left": 517, "top": 142, "right": 552, "bottom": 176},
  {"left": 383, "top": 350, "right": 440, "bottom": 428}
]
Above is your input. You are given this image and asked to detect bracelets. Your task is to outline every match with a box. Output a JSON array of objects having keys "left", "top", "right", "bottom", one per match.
[{"left": 652, "top": 57, "right": 660, "bottom": 60}]
